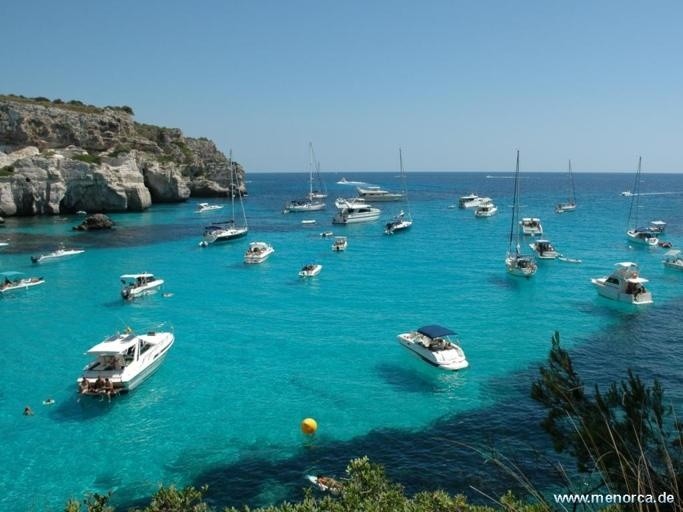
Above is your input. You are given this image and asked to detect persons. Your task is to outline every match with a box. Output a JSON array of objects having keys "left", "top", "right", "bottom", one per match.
[
  {"left": 245, "top": 249, "right": 251, "bottom": 257},
  {"left": 22, "top": 405, "right": 34, "bottom": 417},
  {"left": 0, "top": 280, "right": 11, "bottom": 290},
  {"left": 30, "top": 254, "right": 41, "bottom": 263},
  {"left": 113, "top": 359, "right": 127, "bottom": 373},
  {"left": 44, "top": 398, "right": 51, "bottom": 404},
  {"left": 160, "top": 291, "right": 174, "bottom": 297},
  {"left": 443, "top": 342, "right": 453, "bottom": 350},
  {"left": 99, "top": 377, "right": 114, "bottom": 403},
  {"left": 542, "top": 242, "right": 547, "bottom": 251},
  {"left": 431, "top": 337, "right": 443, "bottom": 351},
  {"left": 88, "top": 375, "right": 104, "bottom": 394},
  {"left": 314, "top": 472, "right": 342, "bottom": 492},
  {"left": 75, "top": 376, "right": 90, "bottom": 395}
]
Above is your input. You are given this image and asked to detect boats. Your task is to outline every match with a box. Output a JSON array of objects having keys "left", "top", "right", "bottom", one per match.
[
  {"left": 74, "top": 328, "right": 175, "bottom": 398},
  {"left": 331, "top": 186, "right": 406, "bottom": 225},
  {"left": 0, "top": 247, "right": 85, "bottom": 294},
  {"left": 243, "top": 231, "right": 348, "bottom": 278},
  {"left": 590, "top": 261, "right": 654, "bottom": 305},
  {"left": 527, "top": 239, "right": 557, "bottom": 260},
  {"left": 661, "top": 247, "right": 683, "bottom": 271},
  {"left": 118, "top": 272, "right": 164, "bottom": 302},
  {"left": 518, "top": 217, "right": 543, "bottom": 237},
  {"left": 395, "top": 324, "right": 469, "bottom": 369},
  {"left": 193, "top": 202, "right": 225, "bottom": 216}
]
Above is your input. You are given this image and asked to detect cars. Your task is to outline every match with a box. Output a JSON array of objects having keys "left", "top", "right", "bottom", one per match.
[{"left": 457, "top": 192, "right": 499, "bottom": 217}]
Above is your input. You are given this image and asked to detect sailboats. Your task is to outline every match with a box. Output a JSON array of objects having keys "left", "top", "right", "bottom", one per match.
[
  {"left": 626, "top": 157, "right": 660, "bottom": 247},
  {"left": 503, "top": 149, "right": 538, "bottom": 277},
  {"left": 281, "top": 141, "right": 329, "bottom": 214},
  {"left": 383, "top": 146, "right": 413, "bottom": 236},
  {"left": 198, "top": 148, "right": 248, "bottom": 248},
  {"left": 555, "top": 158, "right": 577, "bottom": 214}
]
